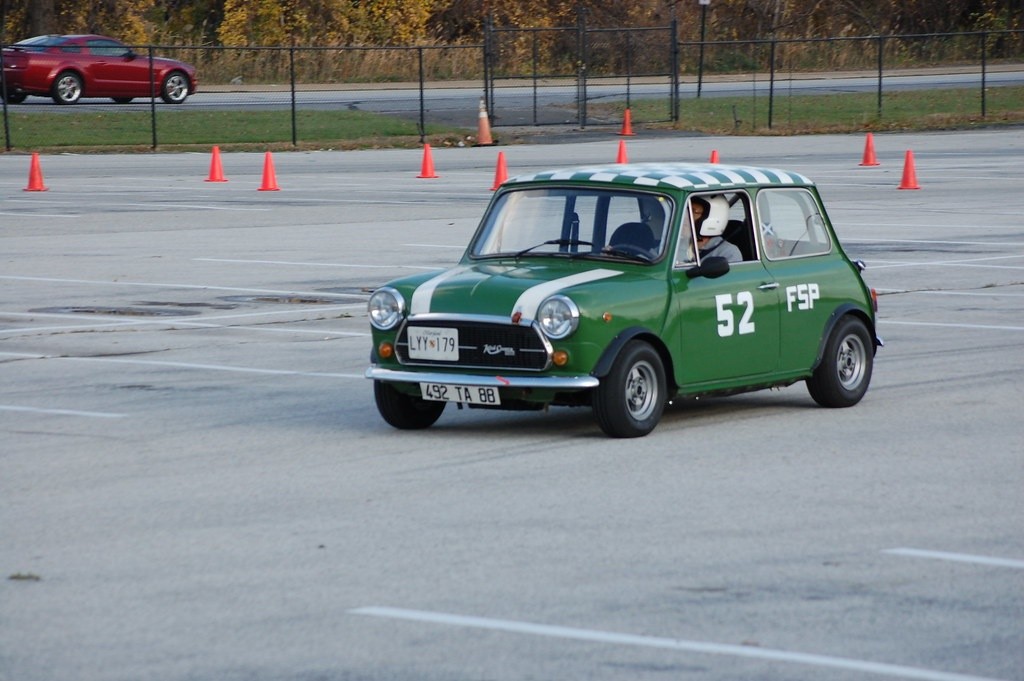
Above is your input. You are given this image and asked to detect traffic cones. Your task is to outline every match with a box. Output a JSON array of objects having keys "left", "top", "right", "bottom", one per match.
[
  {"left": 467, "top": 96, "right": 500, "bottom": 147},
  {"left": 490, "top": 152, "right": 510, "bottom": 192},
  {"left": 203, "top": 144, "right": 229, "bottom": 183},
  {"left": 616, "top": 140, "right": 628, "bottom": 164},
  {"left": 24, "top": 151, "right": 50, "bottom": 191},
  {"left": 257, "top": 151, "right": 280, "bottom": 192},
  {"left": 897, "top": 150, "right": 919, "bottom": 190},
  {"left": 859, "top": 131, "right": 882, "bottom": 168},
  {"left": 415, "top": 144, "right": 440, "bottom": 178},
  {"left": 710, "top": 149, "right": 720, "bottom": 164},
  {"left": 616, "top": 110, "right": 637, "bottom": 137}
]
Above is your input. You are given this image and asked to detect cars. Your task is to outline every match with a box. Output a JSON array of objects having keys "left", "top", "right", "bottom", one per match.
[{"left": 362, "top": 158, "right": 885, "bottom": 440}]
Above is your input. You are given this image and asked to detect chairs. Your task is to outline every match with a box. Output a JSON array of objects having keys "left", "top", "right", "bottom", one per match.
[
  {"left": 609, "top": 222, "right": 651, "bottom": 255},
  {"left": 721, "top": 220, "right": 753, "bottom": 261}
]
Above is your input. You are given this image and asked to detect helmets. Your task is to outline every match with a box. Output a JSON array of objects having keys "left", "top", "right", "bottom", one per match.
[{"left": 689, "top": 193, "right": 731, "bottom": 237}]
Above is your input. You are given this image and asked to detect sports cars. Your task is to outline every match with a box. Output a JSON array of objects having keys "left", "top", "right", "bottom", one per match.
[{"left": 0, "top": 32, "right": 200, "bottom": 105}]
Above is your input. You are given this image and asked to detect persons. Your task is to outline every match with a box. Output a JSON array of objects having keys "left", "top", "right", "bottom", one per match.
[{"left": 601, "top": 194, "right": 742, "bottom": 266}]
